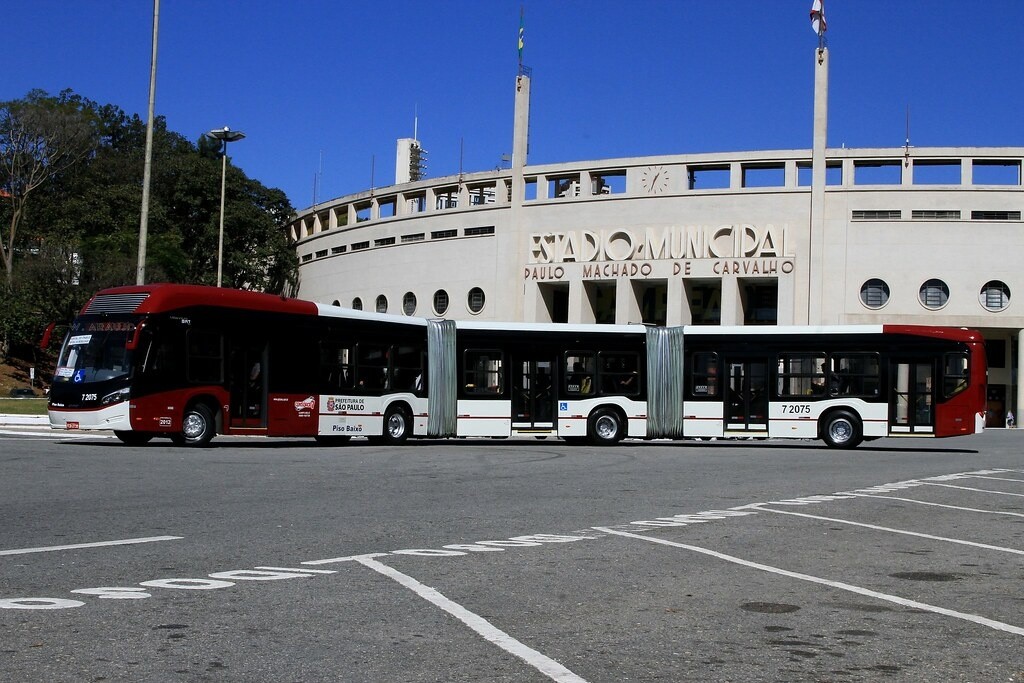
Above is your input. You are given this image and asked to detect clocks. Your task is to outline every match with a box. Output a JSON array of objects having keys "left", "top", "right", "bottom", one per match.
[{"left": 642, "top": 165, "right": 670, "bottom": 194}]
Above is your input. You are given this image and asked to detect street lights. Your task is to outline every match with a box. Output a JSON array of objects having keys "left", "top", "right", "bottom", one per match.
[{"left": 206, "top": 127, "right": 246, "bottom": 288}]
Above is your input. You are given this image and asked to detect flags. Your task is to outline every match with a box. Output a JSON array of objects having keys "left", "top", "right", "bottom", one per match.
[
  {"left": 810, "top": 0, "right": 827, "bottom": 36},
  {"left": 518, "top": 25, "right": 523, "bottom": 60}
]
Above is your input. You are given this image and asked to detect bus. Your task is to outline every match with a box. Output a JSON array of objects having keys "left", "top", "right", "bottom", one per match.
[{"left": 41, "top": 281, "right": 987, "bottom": 448}]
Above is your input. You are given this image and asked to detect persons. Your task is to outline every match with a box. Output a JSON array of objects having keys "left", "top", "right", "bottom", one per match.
[
  {"left": 569, "top": 362, "right": 585, "bottom": 390},
  {"left": 603, "top": 359, "right": 638, "bottom": 394},
  {"left": 944, "top": 369, "right": 969, "bottom": 399},
  {"left": 813, "top": 363, "right": 838, "bottom": 392},
  {"left": 1007, "top": 409, "right": 1014, "bottom": 427}
]
